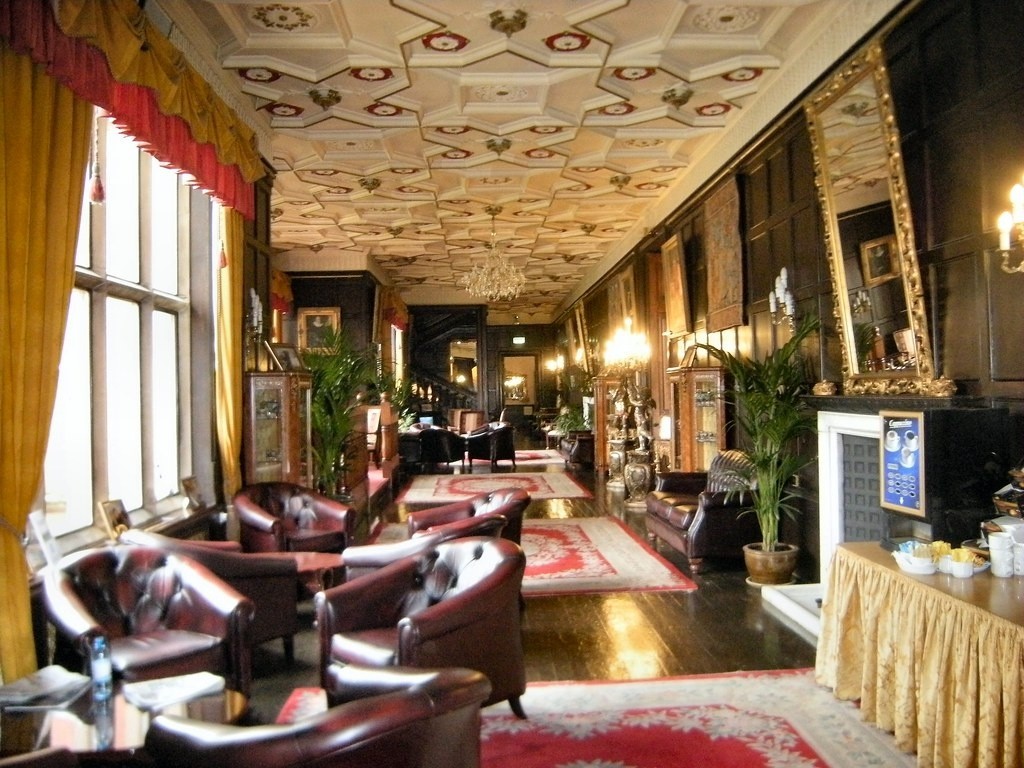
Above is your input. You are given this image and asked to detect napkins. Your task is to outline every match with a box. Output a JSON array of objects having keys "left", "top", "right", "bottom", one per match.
[{"left": 123, "top": 670, "right": 225, "bottom": 713}]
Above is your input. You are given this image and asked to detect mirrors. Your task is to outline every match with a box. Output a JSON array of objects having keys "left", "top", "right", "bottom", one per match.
[
  {"left": 504, "top": 373, "right": 528, "bottom": 400},
  {"left": 503, "top": 355, "right": 536, "bottom": 406},
  {"left": 804, "top": 40, "right": 937, "bottom": 382}
]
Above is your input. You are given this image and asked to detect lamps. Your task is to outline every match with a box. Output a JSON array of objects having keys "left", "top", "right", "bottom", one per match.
[
  {"left": 458, "top": 206, "right": 528, "bottom": 302},
  {"left": 603, "top": 315, "right": 651, "bottom": 382},
  {"left": 766, "top": 265, "right": 802, "bottom": 332},
  {"left": 996, "top": 173, "right": 1024, "bottom": 275}
]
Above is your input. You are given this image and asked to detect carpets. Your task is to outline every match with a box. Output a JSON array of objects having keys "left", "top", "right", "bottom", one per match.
[
  {"left": 275, "top": 667, "right": 919, "bottom": 768},
  {"left": 394, "top": 470, "right": 594, "bottom": 504},
  {"left": 437, "top": 448, "right": 570, "bottom": 468},
  {"left": 360, "top": 515, "right": 699, "bottom": 597}
]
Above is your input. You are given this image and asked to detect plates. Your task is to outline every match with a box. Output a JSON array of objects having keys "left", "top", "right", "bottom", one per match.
[{"left": 884, "top": 442, "right": 918, "bottom": 468}]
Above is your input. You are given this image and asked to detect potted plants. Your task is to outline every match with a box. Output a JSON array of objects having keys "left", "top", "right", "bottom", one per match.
[{"left": 695, "top": 308, "right": 825, "bottom": 588}]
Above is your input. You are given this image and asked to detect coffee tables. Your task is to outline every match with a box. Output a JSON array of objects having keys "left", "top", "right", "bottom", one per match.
[{"left": 250, "top": 551, "right": 346, "bottom": 627}]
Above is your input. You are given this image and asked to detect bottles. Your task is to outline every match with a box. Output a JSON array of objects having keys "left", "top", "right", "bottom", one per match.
[
  {"left": 88, "top": 636, "right": 114, "bottom": 700},
  {"left": 95, "top": 702, "right": 114, "bottom": 751}
]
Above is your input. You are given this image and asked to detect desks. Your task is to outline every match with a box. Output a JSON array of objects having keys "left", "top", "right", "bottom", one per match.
[{"left": 814, "top": 541, "right": 1024, "bottom": 768}]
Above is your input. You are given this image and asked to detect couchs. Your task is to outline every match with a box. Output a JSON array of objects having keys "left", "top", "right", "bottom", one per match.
[
  {"left": 397, "top": 432, "right": 423, "bottom": 468},
  {"left": 527, "top": 408, "right": 570, "bottom": 450},
  {"left": 644, "top": 448, "right": 777, "bottom": 574},
  {"left": 41, "top": 545, "right": 256, "bottom": 703},
  {"left": 231, "top": 480, "right": 356, "bottom": 599},
  {"left": 340, "top": 514, "right": 508, "bottom": 581},
  {"left": 410, "top": 423, "right": 445, "bottom": 434},
  {"left": 144, "top": 661, "right": 492, "bottom": 768},
  {"left": 313, "top": 534, "right": 527, "bottom": 721},
  {"left": 419, "top": 428, "right": 466, "bottom": 469},
  {"left": 407, "top": 487, "right": 532, "bottom": 547},
  {"left": 467, "top": 421, "right": 517, "bottom": 470},
  {"left": 561, "top": 429, "right": 596, "bottom": 469},
  {"left": 118, "top": 530, "right": 297, "bottom": 660}
]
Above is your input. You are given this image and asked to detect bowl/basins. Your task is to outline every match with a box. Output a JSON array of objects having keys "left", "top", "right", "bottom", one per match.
[{"left": 973, "top": 561, "right": 990, "bottom": 573}]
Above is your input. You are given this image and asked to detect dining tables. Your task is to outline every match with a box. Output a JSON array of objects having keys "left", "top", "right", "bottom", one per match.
[{"left": 0, "top": 665, "right": 248, "bottom": 760}]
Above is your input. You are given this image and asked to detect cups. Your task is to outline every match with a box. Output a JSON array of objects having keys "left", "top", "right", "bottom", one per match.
[
  {"left": 901, "top": 448, "right": 915, "bottom": 464},
  {"left": 989, "top": 532, "right": 1024, "bottom": 578},
  {"left": 895, "top": 551, "right": 973, "bottom": 578},
  {"left": 886, "top": 431, "right": 900, "bottom": 448},
  {"left": 903, "top": 430, "right": 918, "bottom": 448}
]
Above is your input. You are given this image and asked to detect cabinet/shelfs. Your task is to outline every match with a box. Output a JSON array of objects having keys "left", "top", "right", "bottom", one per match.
[{"left": 666, "top": 366, "right": 727, "bottom": 472}]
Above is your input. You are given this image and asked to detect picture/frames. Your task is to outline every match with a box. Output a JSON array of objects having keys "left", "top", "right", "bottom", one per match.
[
  {"left": 264, "top": 340, "right": 285, "bottom": 371},
  {"left": 298, "top": 307, "right": 342, "bottom": 357},
  {"left": 180, "top": 476, "right": 207, "bottom": 511},
  {"left": 660, "top": 228, "right": 692, "bottom": 339},
  {"left": 272, "top": 343, "right": 307, "bottom": 371},
  {"left": 28, "top": 510, "right": 64, "bottom": 568},
  {"left": 97, "top": 498, "right": 137, "bottom": 543},
  {"left": 859, "top": 233, "right": 902, "bottom": 291}
]
